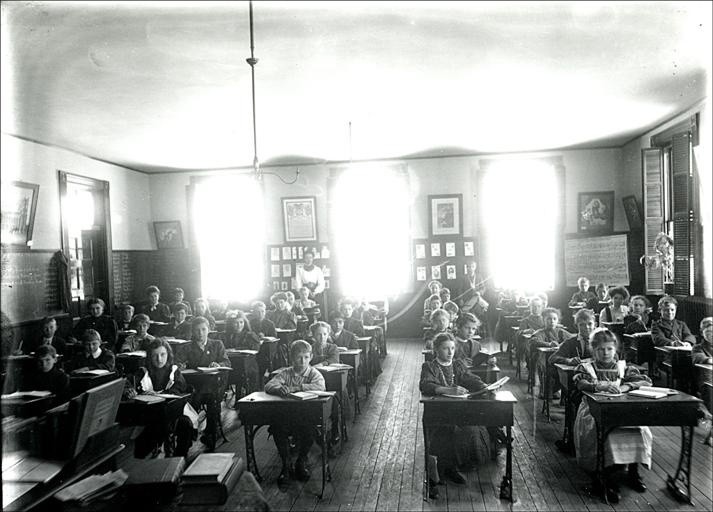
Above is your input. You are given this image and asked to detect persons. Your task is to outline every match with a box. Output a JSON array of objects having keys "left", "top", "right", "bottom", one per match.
[
  {"left": 456, "top": 259, "right": 488, "bottom": 336},
  {"left": 2, "top": 275, "right": 713, "bottom": 505},
  {"left": 158, "top": 228, "right": 181, "bottom": 248},
  {"left": 292, "top": 250, "right": 326, "bottom": 311},
  {"left": 583, "top": 197, "right": 608, "bottom": 228}
]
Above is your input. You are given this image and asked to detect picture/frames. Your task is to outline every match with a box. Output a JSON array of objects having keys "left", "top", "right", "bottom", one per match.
[
  {"left": 152, "top": 219, "right": 186, "bottom": 248},
  {"left": 1, "top": 180, "right": 40, "bottom": 251},
  {"left": 623, "top": 195, "right": 642, "bottom": 229},
  {"left": 577, "top": 190, "right": 615, "bottom": 234},
  {"left": 429, "top": 193, "right": 463, "bottom": 236},
  {"left": 281, "top": 196, "right": 318, "bottom": 244}
]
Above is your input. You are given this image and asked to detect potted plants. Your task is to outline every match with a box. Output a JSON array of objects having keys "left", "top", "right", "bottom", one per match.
[{"left": 640, "top": 233, "right": 676, "bottom": 297}]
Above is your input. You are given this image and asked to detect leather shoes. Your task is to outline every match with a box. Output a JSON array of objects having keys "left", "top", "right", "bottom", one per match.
[
  {"left": 278, "top": 461, "right": 310, "bottom": 482},
  {"left": 626, "top": 469, "right": 646, "bottom": 492},
  {"left": 444, "top": 464, "right": 467, "bottom": 484},
  {"left": 425, "top": 479, "right": 439, "bottom": 499},
  {"left": 328, "top": 431, "right": 340, "bottom": 458},
  {"left": 592, "top": 479, "right": 621, "bottom": 503}
]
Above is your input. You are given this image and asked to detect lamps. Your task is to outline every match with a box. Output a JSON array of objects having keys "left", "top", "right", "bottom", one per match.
[{"left": 232, "top": 1, "right": 300, "bottom": 184}]
